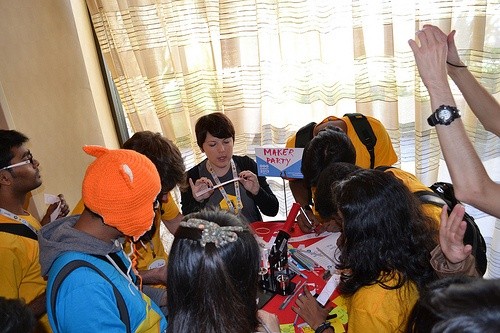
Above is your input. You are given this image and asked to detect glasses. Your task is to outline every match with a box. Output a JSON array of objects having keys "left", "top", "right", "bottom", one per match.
[
  {"left": 0, "top": 154, "right": 33, "bottom": 171},
  {"left": 334, "top": 216, "right": 345, "bottom": 226}
]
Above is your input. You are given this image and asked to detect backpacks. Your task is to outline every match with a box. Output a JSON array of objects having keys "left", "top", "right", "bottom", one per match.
[{"left": 413, "top": 182, "right": 487, "bottom": 278}]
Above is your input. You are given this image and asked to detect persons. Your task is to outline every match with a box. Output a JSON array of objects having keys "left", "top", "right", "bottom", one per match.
[
  {"left": 408, "top": 24, "right": 500, "bottom": 221},
  {"left": 407, "top": 204, "right": 500, "bottom": 333},
  {"left": 0, "top": 129, "right": 70, "bottom": 333},
  {"left": 315, "top": 163, "right": 452, "bottom": 277},
  {"left": 179, "top": 112, "right": 279, "bottom": 224},
  {"left": 285, "top": 112, "right": 398, "bottom": 236},
  {"left": 71, "top": 130, "right": 188, "bottom": 318},
  {"left": 291, "top": 170, "right": 437, "bottom": 333},
  {"left": 38, "top": 144, "right": 167, "bottom": 333},
  {"left": 166, "top": 207, "right": 281, "bottom": 333}
]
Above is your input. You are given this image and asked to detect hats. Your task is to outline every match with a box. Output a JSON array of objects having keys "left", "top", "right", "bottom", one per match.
[{"left": 83, "top": 146, "right": 162, "bottom": 241}]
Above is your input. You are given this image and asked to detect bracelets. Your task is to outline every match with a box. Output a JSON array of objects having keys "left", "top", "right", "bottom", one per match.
[{"left": 315, "top": 321, "right": 334, "bottom": 332}]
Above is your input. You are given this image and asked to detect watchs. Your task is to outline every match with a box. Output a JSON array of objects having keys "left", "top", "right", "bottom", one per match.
[{"left": 427, "top": 104, "right": 460, "bottom": 128}]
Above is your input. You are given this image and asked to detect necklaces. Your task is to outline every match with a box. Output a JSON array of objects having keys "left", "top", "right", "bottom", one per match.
[
  {"left": 0, "top": 208, "right": 39, "bottom": 236},
  {"left": 206, "top": 159, "right": 242, "bottom": 213}
]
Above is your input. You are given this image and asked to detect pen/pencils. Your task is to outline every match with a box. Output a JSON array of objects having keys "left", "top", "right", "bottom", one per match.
[
  {"left": 280, "top": 247, "right": 342, "bottom": 328},
  {"left": 300, "top": 206, "right": 316, "bottom": 232}
]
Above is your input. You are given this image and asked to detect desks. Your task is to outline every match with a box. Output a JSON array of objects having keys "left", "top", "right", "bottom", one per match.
[{"left": 249, "top": 220, "right": 351, "bottom": 333}]
